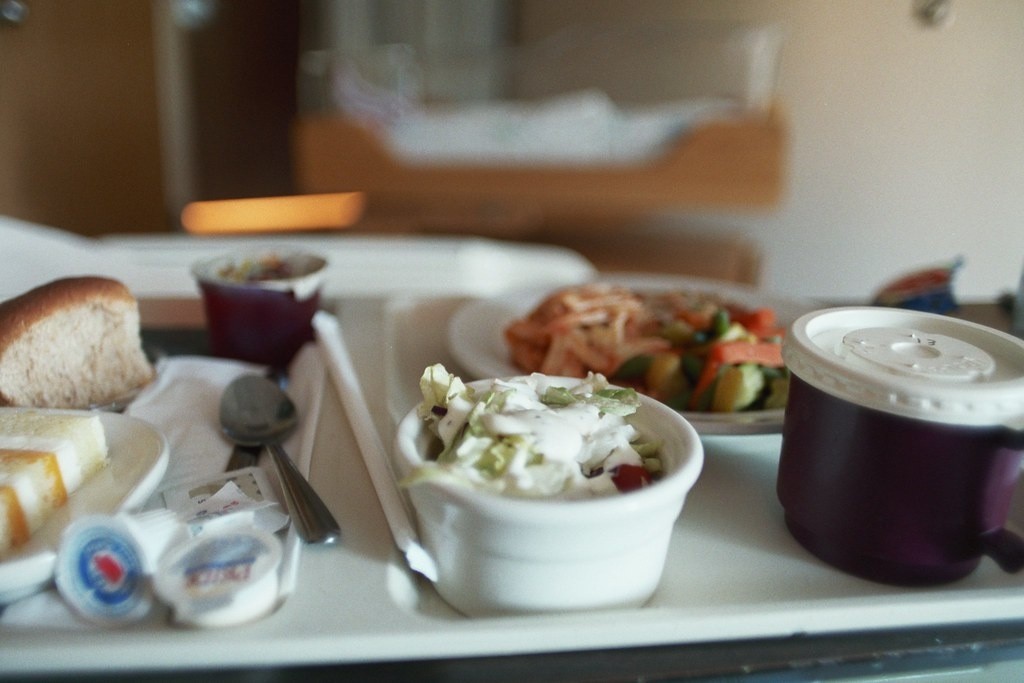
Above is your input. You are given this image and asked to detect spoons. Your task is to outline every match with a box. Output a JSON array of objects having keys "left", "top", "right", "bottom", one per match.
[{"left": 217, "top": 373, "right": 342, "bottom": 548}]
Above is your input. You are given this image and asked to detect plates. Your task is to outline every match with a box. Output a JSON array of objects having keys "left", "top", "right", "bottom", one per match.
[
  {"left": 444, "top": 273, "right": 838, "bottom": 434},
  {"left": 0, "top": 405, "right": 171, "bottom": 605}
]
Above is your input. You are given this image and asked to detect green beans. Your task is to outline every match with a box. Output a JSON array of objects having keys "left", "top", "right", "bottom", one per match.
[{"left": 619, "top": 308, "right": 787, "bottom": 412}]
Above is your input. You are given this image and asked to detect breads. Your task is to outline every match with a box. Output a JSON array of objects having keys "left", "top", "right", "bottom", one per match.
[
  {"left": 0, "top": 404, "right": 110, "bottom": 563},
  {"left": 0, "top": 275, "right": 153, "bottom": 410}
]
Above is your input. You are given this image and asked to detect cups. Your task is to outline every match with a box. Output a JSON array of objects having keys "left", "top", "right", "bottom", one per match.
[
  {"left": 777, "top": 304, "right": 1023, "bottom": 589},
  {"left": 393, "top": 375, "right": 704, "bottom": 620},
  {"left": 188, "top": 246, "right": 334, "bottom": 371}
]
